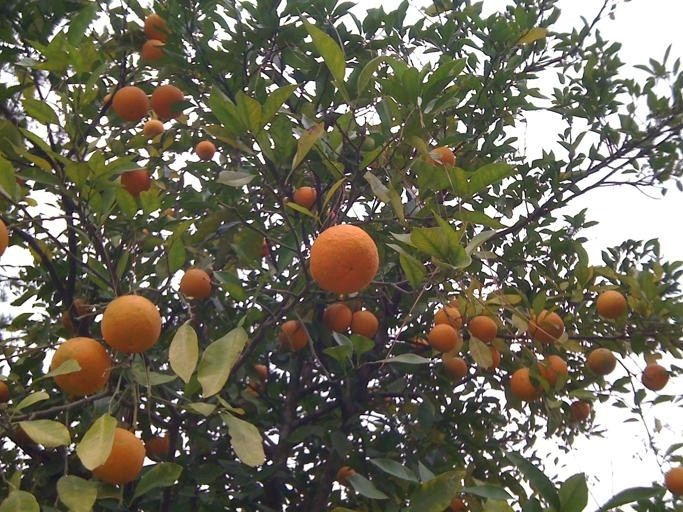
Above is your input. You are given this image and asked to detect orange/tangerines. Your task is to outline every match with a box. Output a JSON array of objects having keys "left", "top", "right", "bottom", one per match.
[{"left": 0, "top": 14, "right": 683, "bottom": 512}]
